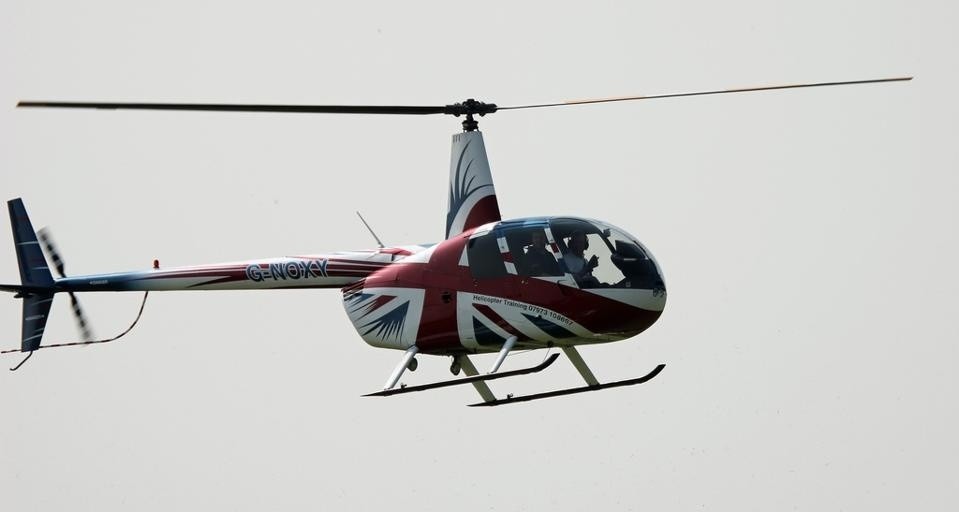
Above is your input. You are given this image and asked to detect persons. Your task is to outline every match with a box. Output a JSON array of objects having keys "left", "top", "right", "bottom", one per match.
[
  {"left": 564, "top": 231, "right": 610, "bottom": 289},
  {"left": 520, "top": 230, "right": 560, "bottom": 275}
]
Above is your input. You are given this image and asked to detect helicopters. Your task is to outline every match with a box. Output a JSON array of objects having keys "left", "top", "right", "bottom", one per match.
[{"left": 0, "top": 78, "right": 913, "bottom": 409}]
